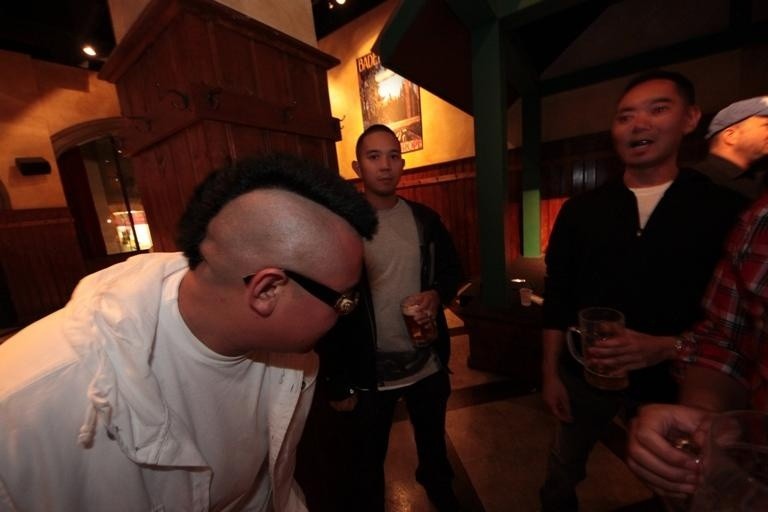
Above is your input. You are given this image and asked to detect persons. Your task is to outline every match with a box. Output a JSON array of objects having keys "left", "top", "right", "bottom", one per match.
[
  {"left": 701, "top": 96, "right": 767, "bottom": 198},
  {"left": 0, "top": 154, "right": 377, "bottom": 512},
  {"left": 539, "top": 73, "right": 749, "bottom": 512},
  {"left": 624, "top": 193, "right": 768, "bottom": 510},
  {"left": 326, "top": 125, "right": 460, "bottom": 510}
]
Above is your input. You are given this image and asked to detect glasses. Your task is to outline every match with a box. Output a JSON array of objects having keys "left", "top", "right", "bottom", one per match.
[{"left": 241, "top": 265, "right": 361, "bottom": 317}]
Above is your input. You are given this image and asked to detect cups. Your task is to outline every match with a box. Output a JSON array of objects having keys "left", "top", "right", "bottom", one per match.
[
  {"left": 398, "top": 294, "right": 439, "bottom": 347},
  {"left": 668, "top": 408, "right": 767, "bottom": 510},
  {"left": 567, "top": 305, "right": 629, "bottom": 390},
  {"left": 519, "top": 288, "right": 533, "bottom": 306}
]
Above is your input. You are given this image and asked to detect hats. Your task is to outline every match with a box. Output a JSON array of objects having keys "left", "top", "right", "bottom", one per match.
[{"left": 704, "top": 95, "right": 767, "bottom": 141}]
